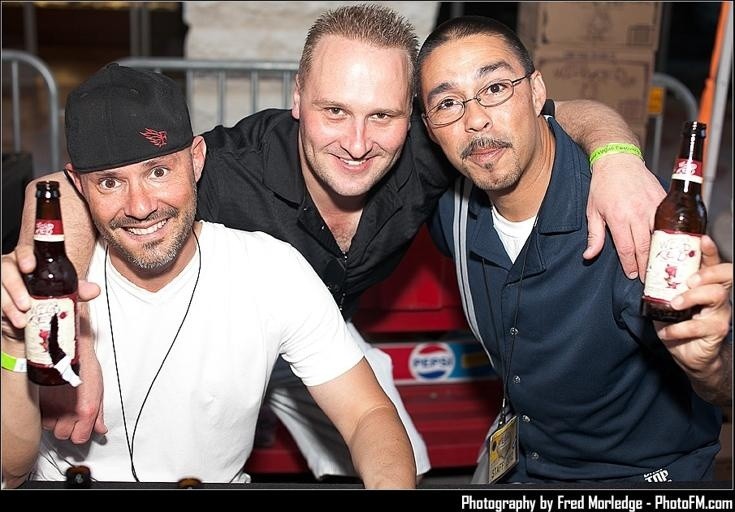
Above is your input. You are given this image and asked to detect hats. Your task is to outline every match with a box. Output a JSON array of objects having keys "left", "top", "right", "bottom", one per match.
[{"left": 62, "top": 63, "right": 195, "bottom": 179}]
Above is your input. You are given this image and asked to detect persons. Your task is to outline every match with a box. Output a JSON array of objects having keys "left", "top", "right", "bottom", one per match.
[
  {"left": 0, "top": 3, "right": 669, "bottom": 491},
  {"left": 414, "top": 11, "right": 735, "bottom": 492},
  {"left": 1, "top": 63, "right": 420, "bottom": 491}
]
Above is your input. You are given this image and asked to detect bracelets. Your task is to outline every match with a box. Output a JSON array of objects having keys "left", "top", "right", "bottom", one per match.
[
  {"left": 1, "top": 351, "right": 28, "bottom": 377},
  {"left": 588, "top": 142, "right": 647, "bottom": 174}
]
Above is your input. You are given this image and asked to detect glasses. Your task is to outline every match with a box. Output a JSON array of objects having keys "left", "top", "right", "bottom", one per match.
[{"left": 423, "top": 72, "right": 533, "bottom": 129}]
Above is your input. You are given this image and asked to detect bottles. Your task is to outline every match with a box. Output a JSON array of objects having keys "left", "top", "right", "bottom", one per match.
[
  {"left": 20, "top": 180, "right": 79, "bottom": 386},
  {"left": 638, "top": 121, "right": 709, "bottom": 322}
]
